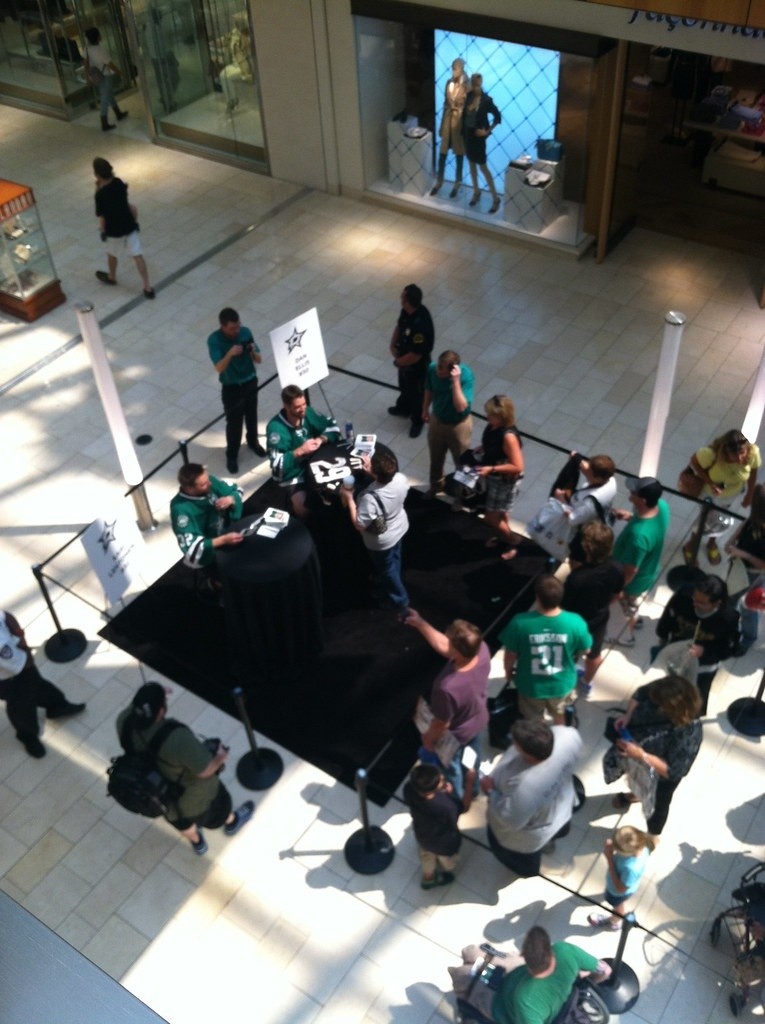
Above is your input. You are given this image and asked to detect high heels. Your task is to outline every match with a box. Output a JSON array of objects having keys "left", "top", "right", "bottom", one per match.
[{"left": 431, "top": 183, "right": 501, "bottom": 214}]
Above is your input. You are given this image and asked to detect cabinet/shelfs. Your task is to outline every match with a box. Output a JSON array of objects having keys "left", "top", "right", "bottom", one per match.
[{"left": 0, "top": 178, "right": 66, "bottom": 322}]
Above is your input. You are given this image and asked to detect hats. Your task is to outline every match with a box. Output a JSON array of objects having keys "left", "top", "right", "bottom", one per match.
[
  {"left": 85, "top": 28, "right": 97, "bottom": 38},
  {"left": 625, "top": 477, "right": 663, "bottom": 497},
  {"left": 94, "top": 159, "right": 112, "bottom": 180},
  {"left": 130, "top": 682, "right": 165, "bottom": 731}
]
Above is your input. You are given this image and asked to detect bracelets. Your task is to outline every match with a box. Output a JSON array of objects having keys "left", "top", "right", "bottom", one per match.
[{"left": 492, "top": 465, "right": 495, "bottom": 474}]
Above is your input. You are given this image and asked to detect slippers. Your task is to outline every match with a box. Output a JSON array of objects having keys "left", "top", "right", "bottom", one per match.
[{"left": 682, "top": 543, "right": 721, "bottom": 567}]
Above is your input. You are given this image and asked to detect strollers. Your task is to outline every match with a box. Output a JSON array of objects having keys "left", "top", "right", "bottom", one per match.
[{"left": 710, "top": 860, "right": 765, "bottom": 1017}]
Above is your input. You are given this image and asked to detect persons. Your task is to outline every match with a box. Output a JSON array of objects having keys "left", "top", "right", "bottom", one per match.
[
  {"left": 385, "top": 283, "right": 434, "bottom": 437},
  {"left": 219, "top": 11, "right": 257, "bottom": 113},
  {"left": 0, "top": 610, "right": 87, "bottom": 759},
  {"left": 141, "top": 8, "right": 181, "bottom": 113},
  {"left": 92, "top": 157, "right": 156, "bottom": 301},
  {"left": 266, "top": 384, "right": 343, "bottom": 483},
  {"left": 459, "top": 73, "right": 502, "bottom": 213},
  {"left": 52, "top": 23, "right": 86, "bottom": 66},
  {"left": 339, "top": 447, "right": 413, "bottom": 624},
  {"left": 429, "top": 58, "right": 470, "bottom": 198},
  {"left": 452, "top": 924, "right": 612, "bottom": 1023},
  {"left": 423, "top": 350, "right": 476, "bottom": 485},
  {"left": 398, "top": 427, "right": 765, "bottom": 929},
  {"left": 114, "top": 682, "right": 255, "bottom": 857},
  {"left": 206, "top": 306, "right": 266, "bottom": 474},
  {"left": 83, "top": 27, "right": 129, "bottom": 132},
  {"left": 472, "top": 393, "right": 525, "bottom": 561},
  {"left": 170, "top": 464, "right": 245, "bottom": 571}
]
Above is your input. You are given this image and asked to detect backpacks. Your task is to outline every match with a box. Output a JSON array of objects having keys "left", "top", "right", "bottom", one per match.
[{"left": 106, "top": 713, "right": 186, "bottom": 818}]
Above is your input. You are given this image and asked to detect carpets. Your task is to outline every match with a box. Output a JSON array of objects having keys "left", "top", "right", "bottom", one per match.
[{"left": 98, "top": 441, "right": 565, "bottom": 807}]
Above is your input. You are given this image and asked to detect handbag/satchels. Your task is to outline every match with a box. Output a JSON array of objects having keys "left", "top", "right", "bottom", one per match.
[
  {"left": 525, "top": 497, "right": 579, "bottom": 564},
  {"left": 365, "top": 490, "right": 387, "bottom": 535},
  {"left": 679, "top": 453, "right": 718, "bottom": 497},
  {"left": 487, "top": 668, "right": 524, "bottom": 734},
  {"left": 643, "top": 639, "right": 699, "bottom": 688},
  {"left": 602, "top": 708, "right": 658, "bottom": 821},
  {"left": 87, "top": 67, "right": 104, "bottom": 84}
]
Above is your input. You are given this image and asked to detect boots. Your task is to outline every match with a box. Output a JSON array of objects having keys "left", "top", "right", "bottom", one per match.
[{"left": 100, "top": 105, "right": 128, "bottom": 131}]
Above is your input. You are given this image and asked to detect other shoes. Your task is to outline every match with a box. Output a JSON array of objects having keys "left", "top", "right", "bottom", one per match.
[
  {"left": 191, "top": 801, "right": 253, "bottom": 854},
  {"left": 388, "top": 407, "right": 424, "bottom": 438},
  {"left": 613, "top": 792, "right": 641, "bottom": 809},
  {"left": 588, "top": 913, "right": 619, "bottom": 931},
  {"left": 95, "top": 270, "right": 155, "bottom": 300},
  {"left": 226, "top": 443, "right": 265, "bottom": 474},
  {"left": 420, "top": 870, "right": 455, "bottom": 890},
  {"left": 733, "top": 637, "right": 758, "bottom": 657},
  {"left": 15, "top": 700, "right": 85, "bottom": 759},
  {"left": 576, "top": 618, "right": 644, "bottom": 694}
]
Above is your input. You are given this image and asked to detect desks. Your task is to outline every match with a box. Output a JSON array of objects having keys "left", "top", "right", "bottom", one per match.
[
  {"left": 308, "top": 444, "right": 381, "bottom": 555},
  {"left": 218, "top": 513, "right": 320, "bottom": 653}
]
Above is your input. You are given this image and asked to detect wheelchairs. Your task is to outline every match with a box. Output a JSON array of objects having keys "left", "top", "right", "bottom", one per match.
[{"left": 456, "top": 943, "right": 610, "bottom": 1024}]
